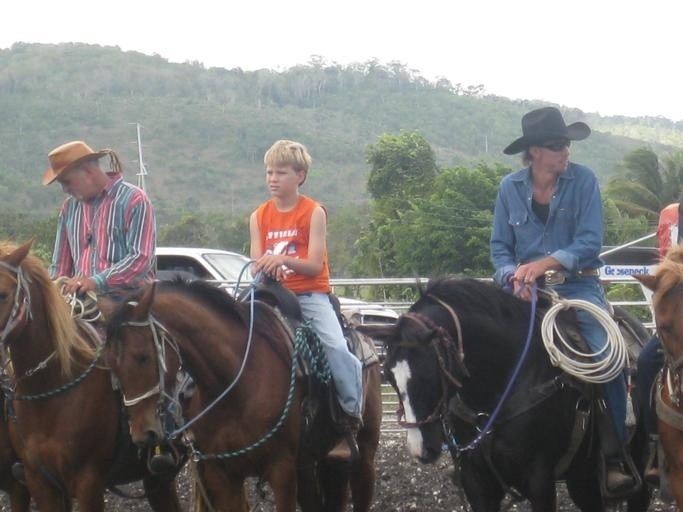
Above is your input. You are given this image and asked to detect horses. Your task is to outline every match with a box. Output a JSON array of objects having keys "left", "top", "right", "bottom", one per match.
[
  {"left": 650, "top": 241, "right": 683, "bottom": 511},
  {"left": 365, "top": 236, "right": 655, "bottom": 512},
  {"left": 1, "top": 236, "right": 140, "bottom": 512},
  {"left": 94, "top": 281, "right": 381, "bottom": 512}
]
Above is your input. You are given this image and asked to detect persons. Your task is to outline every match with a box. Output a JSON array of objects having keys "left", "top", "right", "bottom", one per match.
[
  {"left": 248, "top": 139, "right": 365, "bottom": 462},
  {"left": 41, "top": 140, "right": 179, "bottom": 482},
  {"left": 487, "top": 107, "right": 636, "bottom": 494},
  {"left": 630, "top": 194, "right": 683, "bottom": 486}
]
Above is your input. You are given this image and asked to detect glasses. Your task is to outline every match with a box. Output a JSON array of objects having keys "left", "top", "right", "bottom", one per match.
[{"left": 538, "top": 139, "right": 572, "bottom": 151}]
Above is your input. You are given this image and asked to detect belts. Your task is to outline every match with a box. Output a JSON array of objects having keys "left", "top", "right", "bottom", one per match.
[{"left": 542, "top": 266, "right": 599, "bottom": 286}]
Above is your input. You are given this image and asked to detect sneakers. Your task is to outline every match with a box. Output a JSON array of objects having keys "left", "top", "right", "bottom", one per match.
[
  {"left": 326, "top": 433, "right": 359, "bottom": 461},
  {"left": 605, "top": 464, "right": 634, "bottom": 493},
  {"left": 152, "top": 445, "right": 184, "bottom": 469},
  {"left": 644, "top": 454, "right": 660, "bottom": 486}
]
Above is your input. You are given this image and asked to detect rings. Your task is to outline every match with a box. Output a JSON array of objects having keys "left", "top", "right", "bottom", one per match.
[{"left": 76, "top": 280, "right": 82, "bottom": 286}]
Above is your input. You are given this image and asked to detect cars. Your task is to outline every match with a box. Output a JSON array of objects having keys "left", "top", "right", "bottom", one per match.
[{"left": 150, "top": 245, "right": 399, "bottom": 361}]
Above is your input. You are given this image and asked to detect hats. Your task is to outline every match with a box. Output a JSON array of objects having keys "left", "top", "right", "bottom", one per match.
[
  {"left": 503, "top": 107, "right": 591, "bottom": 155},
  {"left": 42, "top": 141, "right": 123, "bottom": 188}
]
